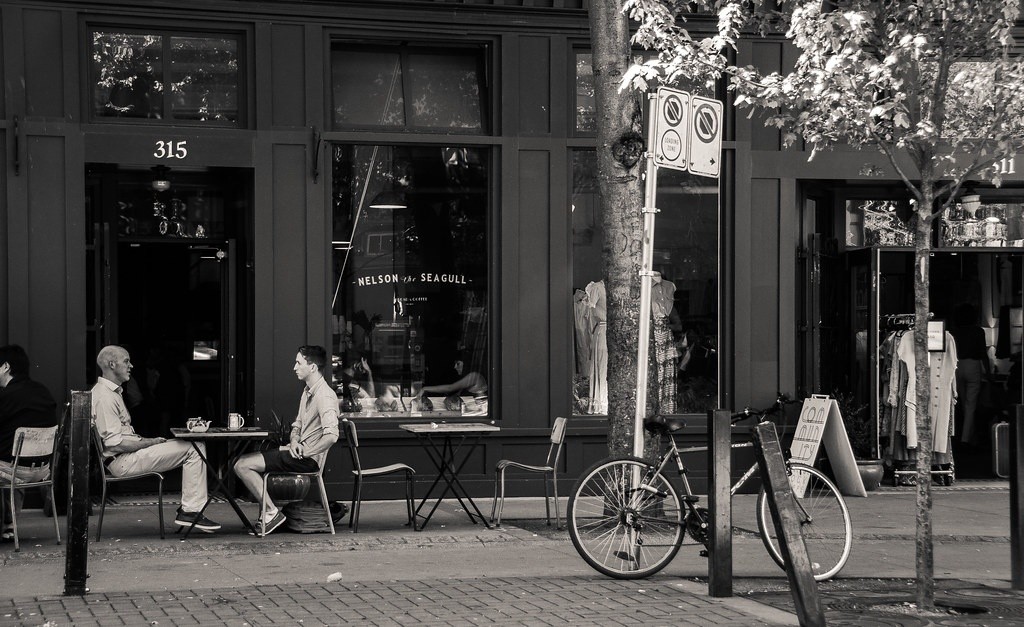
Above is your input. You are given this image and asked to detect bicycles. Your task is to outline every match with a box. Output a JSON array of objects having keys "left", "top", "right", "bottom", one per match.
[{"left": 565, "top": 393, "right": 852, "bottom": 580}]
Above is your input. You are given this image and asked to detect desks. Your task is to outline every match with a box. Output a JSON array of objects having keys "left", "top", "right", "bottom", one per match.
[
  {"left": 398, "top": 423, "right": 501, "bottom": 531},
  {"left": 171, "top": 428, "right": 269, "bottom": 540}
]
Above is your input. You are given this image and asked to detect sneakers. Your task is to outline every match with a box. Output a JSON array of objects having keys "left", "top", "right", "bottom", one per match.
[
  {"left": 247, "top": 510, "right": 286, "bottom": 537},
  {"left": 175, "top": 505, "right": 215, "bottom": 534},
  {"left": 174, "top": 508, "right": 222, "bottom": 530}
]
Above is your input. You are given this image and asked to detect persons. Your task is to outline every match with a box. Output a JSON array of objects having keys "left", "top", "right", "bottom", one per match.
[
  {"left": 956, "top": 319, "right": 991, "bottom": 445},
  {"left": 419, "top": 350, "right": 485, "bottom": 399},
  {"left": 229, "top": 346, "right": 340, "bottom": 536},
  {"left": 91, "top": 346, "right": 222, "bottom": 533},
  {"left": 650, "top": 270, "right": 684, "bottom": 415},
  {"left": 0, "top": 342, "right": 57, "bottom": 541},
  {"left": 587, "top": 277, "right": 610, "bottom": 416},
  {"left": 341, "top": 349, "right": 376, "bottom": 398}
]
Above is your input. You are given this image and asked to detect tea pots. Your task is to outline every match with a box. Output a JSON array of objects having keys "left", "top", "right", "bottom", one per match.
[{"left": 187, "top": 417, "right": 212, "bottom": 433}]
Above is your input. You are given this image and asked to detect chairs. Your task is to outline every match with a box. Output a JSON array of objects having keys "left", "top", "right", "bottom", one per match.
[
  {"left": 342, "top": 417, "right": 417, "bottom": 534},
  {"left": 259, "top": 445, "right": 336, "bottom": 537},
  {"left": 0, "top": 424, "right": 62, "bottom": 553},
  {"left": 490, "top": 417, "right": 568, "bottom": 531},
  {"left": 91, "top": 425, "right": 165, "bottom": 541}
]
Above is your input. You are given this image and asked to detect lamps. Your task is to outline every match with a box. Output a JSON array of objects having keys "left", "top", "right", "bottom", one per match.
[{"left": 368, "top": 144, "right": 408, "bottom": 209}]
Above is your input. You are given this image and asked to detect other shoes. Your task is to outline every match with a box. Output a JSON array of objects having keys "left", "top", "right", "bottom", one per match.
[{"left": 1, "top": 521, "right": 16, "bottom": 541}]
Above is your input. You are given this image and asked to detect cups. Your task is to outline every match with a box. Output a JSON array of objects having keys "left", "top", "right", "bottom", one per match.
[
  {"left": 228, "top": 412, "right": 244, "bottom": 430},
  {"left": 187, "top": 417, "right": 198, "bottom": 430}
]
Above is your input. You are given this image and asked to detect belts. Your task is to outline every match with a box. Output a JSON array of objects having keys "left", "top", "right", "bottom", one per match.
[{"left": 103, "top": 451, "right": 122, "bottom": 467}]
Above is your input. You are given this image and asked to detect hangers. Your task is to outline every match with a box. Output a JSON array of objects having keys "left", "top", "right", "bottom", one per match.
[{"left": 885, "top": 312, "right": 932, "bottom": 337}]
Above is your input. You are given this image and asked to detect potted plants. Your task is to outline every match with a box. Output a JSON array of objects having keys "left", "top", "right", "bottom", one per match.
[{"left": 829, "top": 388, "right": 885, "bottom": 492}]
